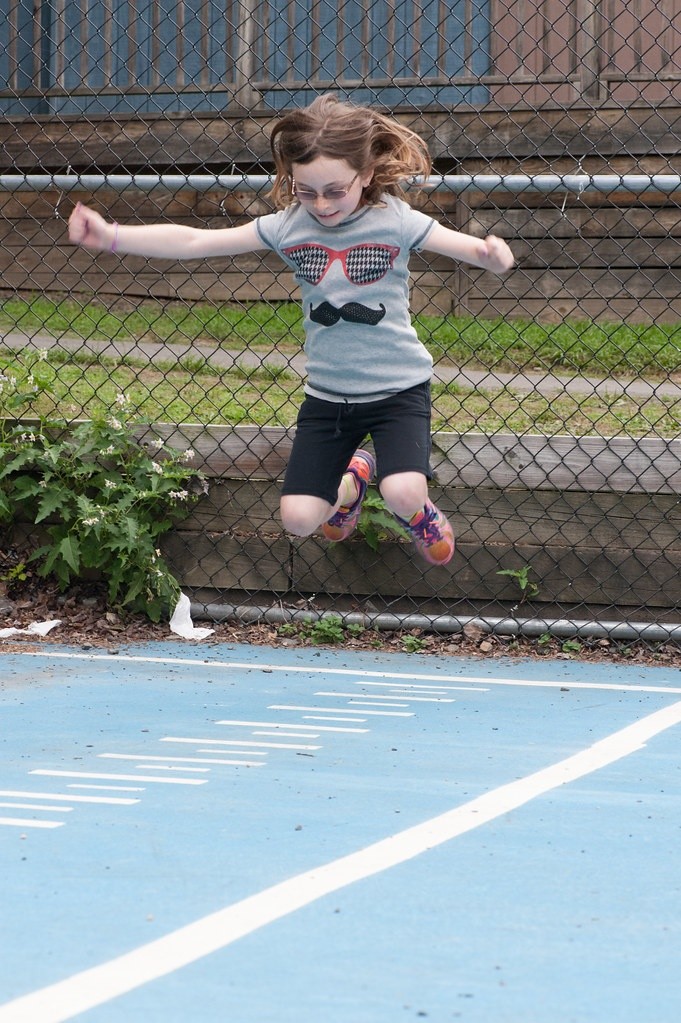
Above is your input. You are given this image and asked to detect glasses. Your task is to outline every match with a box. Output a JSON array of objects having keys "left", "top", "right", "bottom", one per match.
[{"left": 292, "top": 171, "right": 362, "bottom": 199}]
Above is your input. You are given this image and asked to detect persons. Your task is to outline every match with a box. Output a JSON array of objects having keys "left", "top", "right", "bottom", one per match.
[{"left": 68, "top": 93, "right": 514, "bottom": 566}]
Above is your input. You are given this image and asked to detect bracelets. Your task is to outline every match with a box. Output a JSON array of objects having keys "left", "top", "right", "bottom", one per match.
[{"left": 112, "top": 221, "right": 118, "bottom": 253}]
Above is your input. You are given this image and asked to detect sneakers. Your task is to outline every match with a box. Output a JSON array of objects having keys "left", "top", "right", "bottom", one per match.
[
  {"left": 322, "top": 449, "right": 375, "bottom": 543},
  {"left": 394, "top": 496, "right": 454, "bottom": 565}
]
